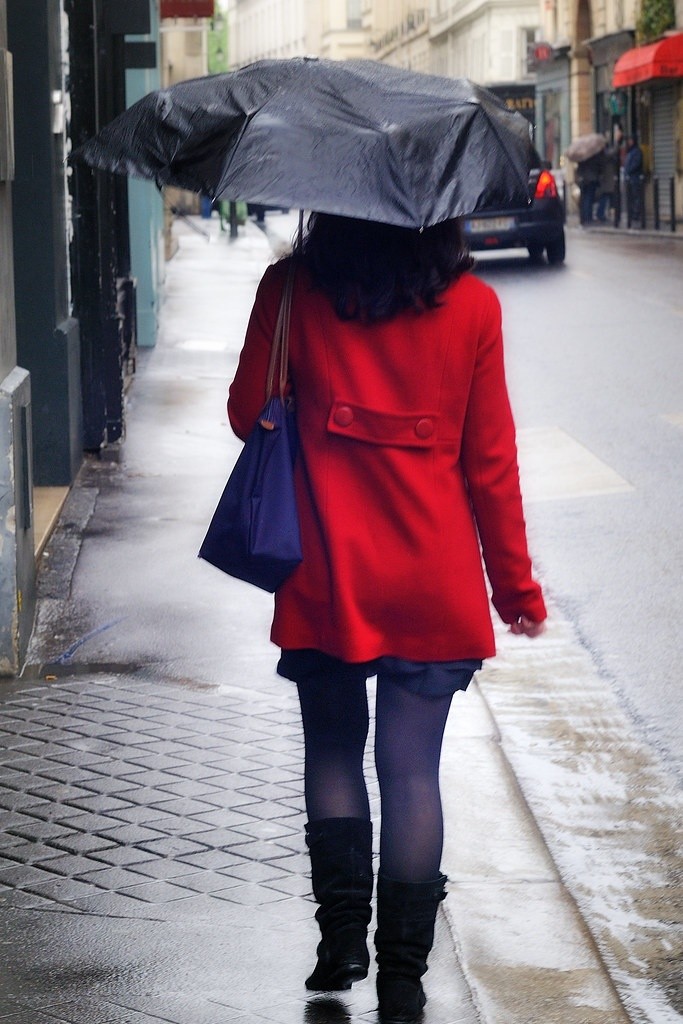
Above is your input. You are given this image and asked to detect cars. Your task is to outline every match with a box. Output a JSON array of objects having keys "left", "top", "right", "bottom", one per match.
[{"left": 457, "top": 148, "right": 566, "bottom": 268}]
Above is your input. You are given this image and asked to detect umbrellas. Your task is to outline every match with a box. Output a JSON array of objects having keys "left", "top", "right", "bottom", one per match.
[
  {"left": 65, "top": 57, "right": 538, "bottom": 246},
  {"left": 567, "top": 134, "right": 608, "bottom": 162}
]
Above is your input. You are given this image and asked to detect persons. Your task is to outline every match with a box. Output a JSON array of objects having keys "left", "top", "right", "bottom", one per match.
[
  {"left": 576, "top": 149, "right": 613, "bottom": 226},
  {"left": 228, "top": 214, "right": 547, "bottom": 1006},
  {"left": 622, "top": 137, "right": 646, "bottom": 230}
]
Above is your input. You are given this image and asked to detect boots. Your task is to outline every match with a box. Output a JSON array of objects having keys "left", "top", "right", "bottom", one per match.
[
  {"left": 305, "top": 817, "right": 374, "bottom": 991},
  {"left": 374, "top": 867, "right": 447, "bottom": 1024}
]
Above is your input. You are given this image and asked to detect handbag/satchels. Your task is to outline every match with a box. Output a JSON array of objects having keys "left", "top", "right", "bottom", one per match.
[{"left": 198, "top": 254, "right": 302, "bottom": 594}]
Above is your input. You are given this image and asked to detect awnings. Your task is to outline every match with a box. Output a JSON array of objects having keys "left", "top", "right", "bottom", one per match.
[{"left": 613, "top": 32, "right": 683, "bottom": 88}]
